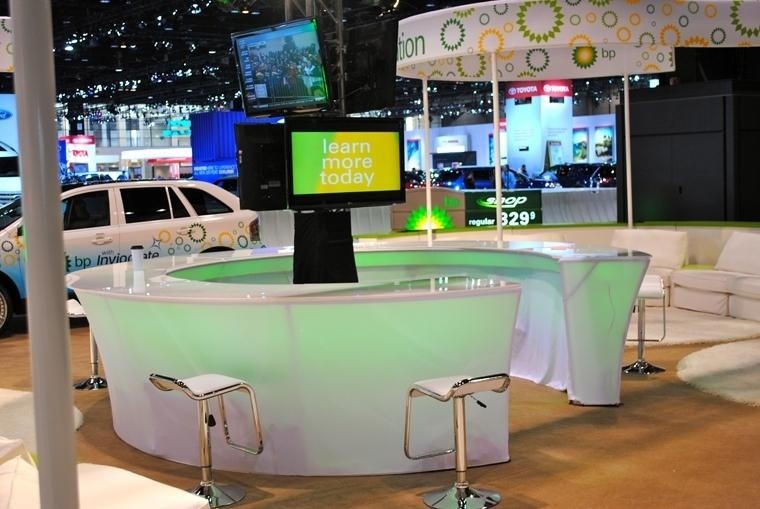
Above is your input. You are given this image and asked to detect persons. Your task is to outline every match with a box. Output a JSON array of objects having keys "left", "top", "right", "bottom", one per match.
[
  {"left": 250, "top": 39, "right": 328, "bottom": 103},
  {"left": 502, "top": 163, "right": 518, "bottom": 190},
  {"left": 68, "top": 162, "right": 76, "bottom": 176},
  {"left": 116, "top": 170, "right": 127, "bottom": 180},
  {"left": 517, "top": 164, "right": 529, "bottom": 176}
]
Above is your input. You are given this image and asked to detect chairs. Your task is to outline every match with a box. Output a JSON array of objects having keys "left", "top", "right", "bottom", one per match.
[
  {"left": 607, "top": 229, "right": 690, "bottom": 307},
  {"left": 671, "top": 230, "right": 760, "bottom": 323}
]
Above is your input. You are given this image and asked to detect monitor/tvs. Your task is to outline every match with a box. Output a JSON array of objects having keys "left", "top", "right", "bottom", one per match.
[
  {"left": 230, "top": 16, "right": 334, "bottom": 117},
  {"left": 284, "top": 116, "right": 407, "bottom": 212},
  {"left": 0, "top": 75, "right": 19, "bottom": 178},
  {"left": 234, "top": 122, "right": 290, "bottom": 211}
]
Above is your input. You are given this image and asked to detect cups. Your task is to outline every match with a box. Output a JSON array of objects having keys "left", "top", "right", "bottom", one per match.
[
  {"left": 130, "top": 245, "right": 145, "bottom": 269},
  {"left": 133, "top": 270, "right": 145, "bottom": 295}
]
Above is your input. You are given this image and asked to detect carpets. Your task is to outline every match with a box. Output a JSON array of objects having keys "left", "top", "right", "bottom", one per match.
[
  {"left": 677, "top": 339, "right": 760, "bottom": 407},
  {"left": 625, "top": 306, "right": 760, "bottom": 347}
]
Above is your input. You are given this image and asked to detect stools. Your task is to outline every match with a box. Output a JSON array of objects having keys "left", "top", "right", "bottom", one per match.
[
  {"left": 66, "top": 298, "right": 108, "bottom": 390},
  {"left": 404, "top": 370, "right": 511, "bottom": 509},
  {"left": 621, "top": 274, "right": 667, "bottom": 376},
  {"left": 148, "top": 373, "right": 263, "bottom": 509}
]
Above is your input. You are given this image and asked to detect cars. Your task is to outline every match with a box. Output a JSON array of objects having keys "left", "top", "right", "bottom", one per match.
[
  {"left": 405, "top": 163, "right": 617, "bottom": 189},
  {"left": 0, "top": 179, "right": 268, "bottom": 341}
]
